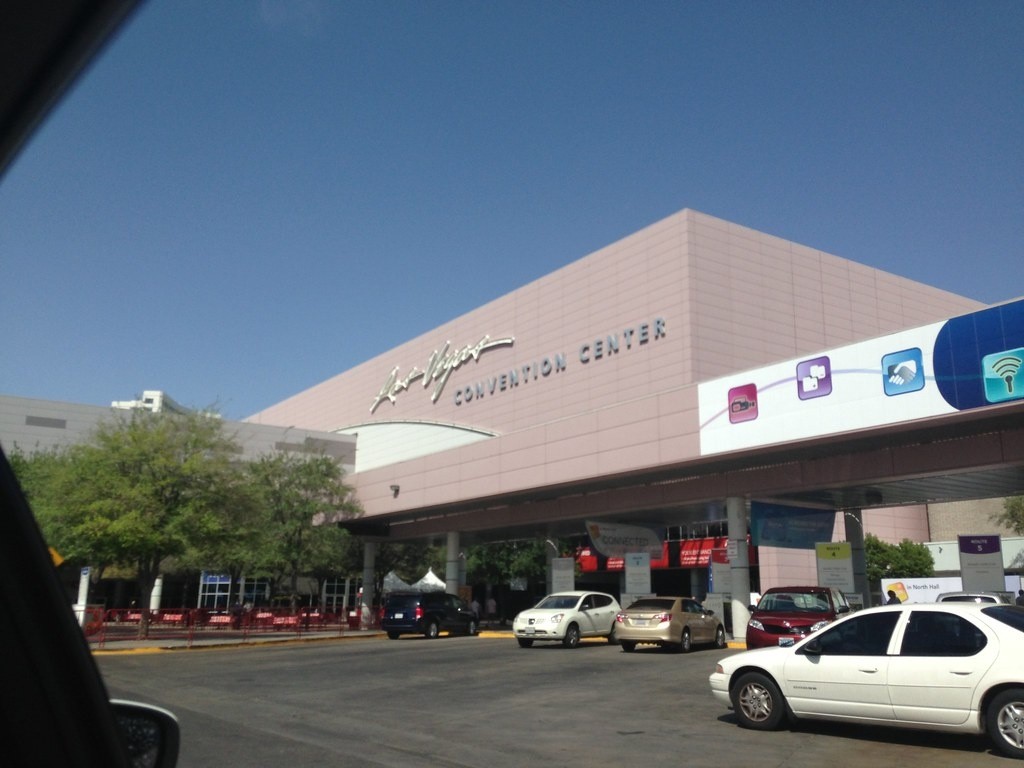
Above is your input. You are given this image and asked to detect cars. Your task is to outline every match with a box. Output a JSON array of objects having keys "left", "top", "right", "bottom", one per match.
[
  {"left": 513, "top": 592, "right": 623, "bottom": 649},
  {"left": 935, "top": 591, "right": 1011, "bottom": 638},
  {"left": 745, "top": 586, "right": 856, "bottom": 651},
  {"left": 615, "top": 596, "right": 726, "bottom": 652},
  {"left": 709, "top": 604, "right": 1024, "bottom": 760},
  {"left": 0, "top": 448, "right": 180, "bottom": 768}
]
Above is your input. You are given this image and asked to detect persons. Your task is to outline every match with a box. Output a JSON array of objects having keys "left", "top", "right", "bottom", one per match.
[
  {"left": 1016, "top": 589, "right": 1024, "bottom": 606},
  {"left": 887, "top": 590, "right": 901, "bottom": 604},
  {"left": 486, "top": 597, "right": 497, "bottom": 623},
  {"left": 471, "top": 599, "right": 480, "bottom": 618}
]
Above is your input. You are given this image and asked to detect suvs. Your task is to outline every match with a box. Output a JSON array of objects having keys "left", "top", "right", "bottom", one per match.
[{"left": 381, "top": 590, "right": 480, "bottom": 640}]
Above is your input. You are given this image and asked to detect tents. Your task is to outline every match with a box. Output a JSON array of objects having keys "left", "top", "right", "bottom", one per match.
[{"left": 359, "top": 566, "right": 446, "bottom": 607}]
